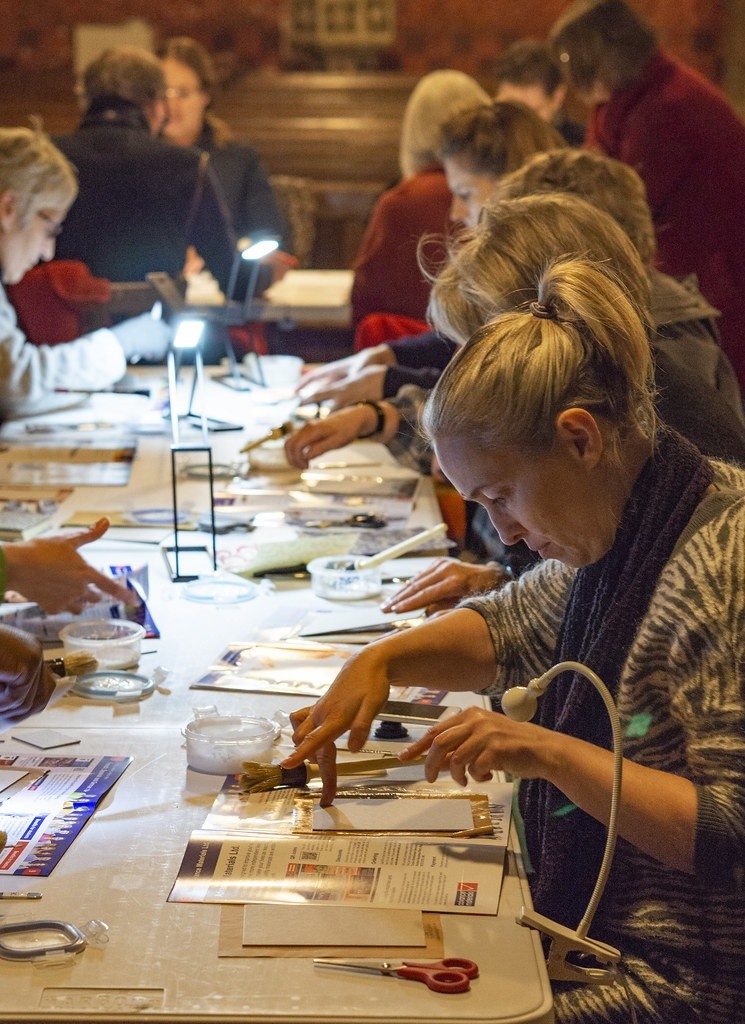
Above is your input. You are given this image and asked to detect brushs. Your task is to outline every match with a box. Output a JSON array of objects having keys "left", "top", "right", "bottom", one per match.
[
  {"left": 242, "top": 419, "right": 296, "bottom": 455},
  {"left": 48, "top": 650, "right": 98, "bottom": 678},
  {"left": 345, "top": 522, "right": 448, "bottom": 582},
  {"left": 237, "top": 752, "right": 459, "bottom": 794}
]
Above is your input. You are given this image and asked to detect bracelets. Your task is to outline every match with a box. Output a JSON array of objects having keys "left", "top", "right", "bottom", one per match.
[{"left": 354, "top": 401, "right": 386, "bottom": 440}]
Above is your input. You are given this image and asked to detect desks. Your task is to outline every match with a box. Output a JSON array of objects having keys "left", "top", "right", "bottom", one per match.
[
  {"left": 183, "top": 269, "right": 353, "bottom": 365},
  {"left": 0, "top": 363, "right": 558, "bottom": 1023}
]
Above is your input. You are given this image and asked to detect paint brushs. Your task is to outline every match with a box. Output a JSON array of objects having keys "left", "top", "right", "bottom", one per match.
[{"left": 52, "top": 387, "right": 158, "bottom": 397}]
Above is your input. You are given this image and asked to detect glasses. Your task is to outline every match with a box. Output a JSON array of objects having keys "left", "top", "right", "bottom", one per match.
[{"left": 36, "top": 210, "right": 63, "bottom": 239}]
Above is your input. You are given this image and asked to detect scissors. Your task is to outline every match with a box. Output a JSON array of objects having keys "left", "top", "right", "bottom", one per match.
[
  {"left": 323, "top": 513, "right": 388, "bottom": 529},
  {"left": 317, "top": 958, "right": 480, "bottom": 995}
]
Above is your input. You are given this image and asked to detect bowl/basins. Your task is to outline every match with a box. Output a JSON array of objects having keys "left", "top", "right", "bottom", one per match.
[
  {"left": 59, "top": 618, "right": 146, "bottom": 670},
  {"left": 180, "top": 705, "right": 291, "bottom": 776},
  {"left": 306, "top": 554, "right": 381, "bottom": 600},
  {"left": 246, "top": 446, "right": 312, "bottom": 486},
  {"left": 245, "top": 354, "right": 304, "bottom": 391}
]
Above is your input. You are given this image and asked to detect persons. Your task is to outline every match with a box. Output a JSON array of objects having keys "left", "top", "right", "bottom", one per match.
[
  {"left": 282, "top": 100, "right": 745, "bottom": 475},
  {"left": 281, "top": 257, "right": 745, "bottom": 1024},
  {"left": 348, "top": 67, "right": 498, "bottom": 349},
  {"left": 0, "top": 517, "right": 141, "bottom": 617},
  {"left": 149, "top": 36, "right": 290, "bottom": 258},
  {"left": 30, "top": 43, "right": 301, "bottom": 300},
  {"left": 547, "top": 0, "right": 744, "bottom": 394},
  {"left": 378, "top": 196, "right": 745, "bottom": 617},
  {"left": 490, "top": 37, "right": 587, "bottom": 147},
  {"left": 0, "top": 126, "right": 174, "bottom": 417}
]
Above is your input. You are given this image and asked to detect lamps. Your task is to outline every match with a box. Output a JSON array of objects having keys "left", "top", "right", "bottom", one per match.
[
  {"left": 165, "top": 317, "right": 245, "bottom": 432},
  {"left": 211, "top": 222, "right": 283, "bottom": 389}
]
